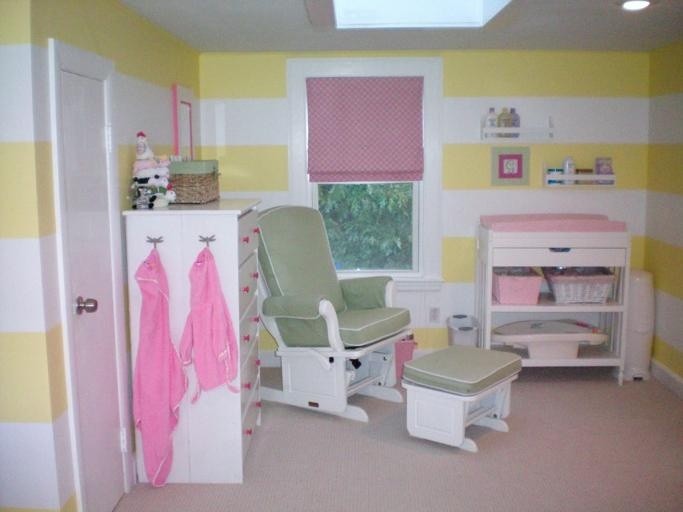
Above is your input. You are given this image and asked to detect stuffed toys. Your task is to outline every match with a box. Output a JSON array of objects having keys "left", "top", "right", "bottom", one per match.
[{"left": 129, "top": 132, "right": 175, "bottom": 208}]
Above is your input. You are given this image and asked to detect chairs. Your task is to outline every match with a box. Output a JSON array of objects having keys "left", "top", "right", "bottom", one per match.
[{"left": 257, "top": 206, "right": 411, "bottom": 424}]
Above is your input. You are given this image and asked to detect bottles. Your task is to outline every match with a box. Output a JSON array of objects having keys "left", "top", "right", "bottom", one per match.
[{"left": 485, "top": 108, "right": 521, "bottom": 137}]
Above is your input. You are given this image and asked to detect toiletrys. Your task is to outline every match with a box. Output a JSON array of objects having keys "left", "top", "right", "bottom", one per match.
[
  {"left": 484, "top": 108, "right": 498, "bottom": 138},
  {"left": 510, "top": 108, "right": 520, "bottom": 139},
  {"left": 498, "top": 107, "right": 511, "bottom": 138}
]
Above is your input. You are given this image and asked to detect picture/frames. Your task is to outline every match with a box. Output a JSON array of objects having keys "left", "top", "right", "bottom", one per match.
[{"left": 491, "top": 147, "right": 529, "bottom": 185}]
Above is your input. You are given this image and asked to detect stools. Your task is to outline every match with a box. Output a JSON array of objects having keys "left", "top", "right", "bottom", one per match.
[{"left": 399, "top": 345, "right": 522, "bottom": 453}]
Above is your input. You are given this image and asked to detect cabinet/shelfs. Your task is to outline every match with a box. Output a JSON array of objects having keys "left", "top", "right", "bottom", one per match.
[
  {"left": 124, "top": 211, "right": 261, "bottom": 484},
  {"left": 478, "top": 230, "right": 631, "bottom": 388},
  {"left": 481, "top": 117, "right": 615, "bottom": 190}
]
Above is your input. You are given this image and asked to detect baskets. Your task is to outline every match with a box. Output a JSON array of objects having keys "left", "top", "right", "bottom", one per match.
[{"left": 542, "top": 266, "right": 616, "bottom": 305}]
